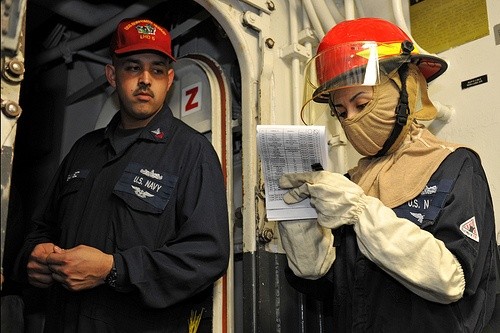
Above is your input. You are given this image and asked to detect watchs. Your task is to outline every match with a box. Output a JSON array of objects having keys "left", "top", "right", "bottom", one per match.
[{"left": 105, "top": 261, "right": 120, "bottom": 289}]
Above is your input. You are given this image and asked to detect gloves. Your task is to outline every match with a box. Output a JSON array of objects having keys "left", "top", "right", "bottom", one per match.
[
  {"left": 278, "top": 170, "right": 465, "bottom": 305},
  {"left": 261, "top": 183, "right": 338, "bottom": 280}
]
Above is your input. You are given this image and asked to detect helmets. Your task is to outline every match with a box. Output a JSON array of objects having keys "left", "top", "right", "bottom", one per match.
[{"left": 311, "top": 18, "right": 448, "bottom": 104}]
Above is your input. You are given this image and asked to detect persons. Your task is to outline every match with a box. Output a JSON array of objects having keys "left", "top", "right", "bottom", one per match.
[
  {"left": 275, "top": 17, "right": 500, "bottom": 333},
  {"left": 9, "top": 18, "right": 233, "bottom": 333}
]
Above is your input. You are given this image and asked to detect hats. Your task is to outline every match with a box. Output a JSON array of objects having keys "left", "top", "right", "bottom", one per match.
[{"left": 111, "top": 18, "right": 176, "bottom": 62}]
